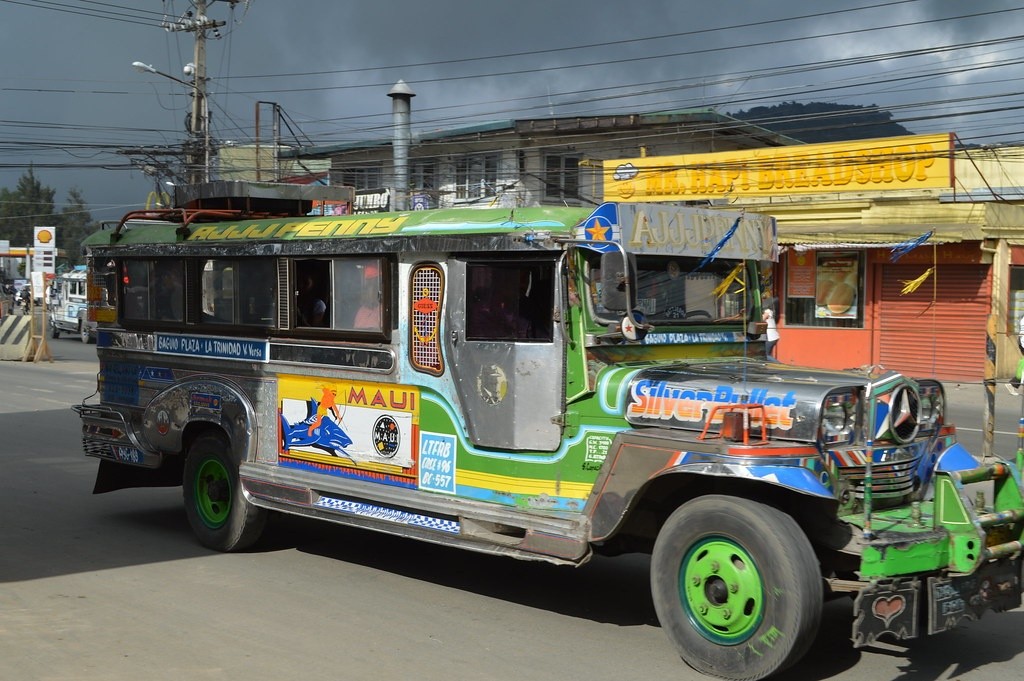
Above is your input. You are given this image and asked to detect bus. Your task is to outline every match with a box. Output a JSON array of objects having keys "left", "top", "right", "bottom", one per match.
[
  {"left": 48, "top": 269, "right": 117, "bottom": 344},
  {"left": 70, "top": 181, "right": 1024, "bottom": 680}
]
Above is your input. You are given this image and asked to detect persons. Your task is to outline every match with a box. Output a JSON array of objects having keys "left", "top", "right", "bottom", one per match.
[
  {"left": 19, "top": 287, "right": 31, "bottom": 311},
  {"left": 761, "top": 296, "right": 783, "bottom": 364},
  {"left": 298, "top": 272, "right": 327, "bottom": 326},
  {"left": 467, "top": 263, "right": 552, "bottom": 337}
]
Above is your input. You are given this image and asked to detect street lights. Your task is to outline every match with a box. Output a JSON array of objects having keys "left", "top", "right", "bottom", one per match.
[{"left": 130, "top": 60, "right": 211, "bottom": 185}]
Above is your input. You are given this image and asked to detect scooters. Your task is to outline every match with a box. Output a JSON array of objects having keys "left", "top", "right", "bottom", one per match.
[{"left": 20, "top": 298, "right": 29, "bottom": 315}]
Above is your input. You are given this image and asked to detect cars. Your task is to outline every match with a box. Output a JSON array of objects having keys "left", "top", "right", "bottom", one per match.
[{"left": 16, "top": 283, "right": 30, "bottom": 306}]
